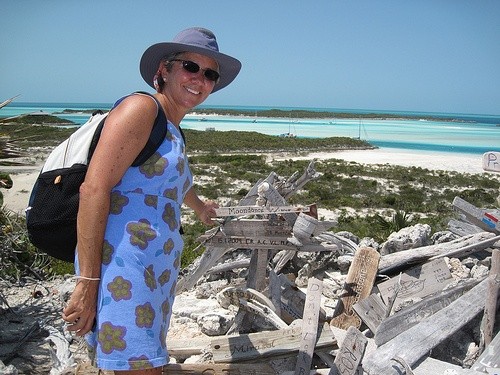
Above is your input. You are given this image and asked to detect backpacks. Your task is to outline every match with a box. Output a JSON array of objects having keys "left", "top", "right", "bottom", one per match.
[{"left": 25, "top": 90, "right": 188, "bottom": 264}]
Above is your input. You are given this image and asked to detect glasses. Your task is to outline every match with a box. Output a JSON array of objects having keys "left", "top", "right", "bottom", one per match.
[{"left": 168, "top": 58, "right": 220, "bottom": 81}]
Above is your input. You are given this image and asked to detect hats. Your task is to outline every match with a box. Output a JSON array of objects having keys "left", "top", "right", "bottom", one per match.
[{"left": 140, "top": 27, "right": 242, "bottom": 94}]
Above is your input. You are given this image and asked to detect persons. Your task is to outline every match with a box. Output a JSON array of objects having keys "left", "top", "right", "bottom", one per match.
[{"left": 60, "top": 26, "right": 244, "bottom": 375}]
